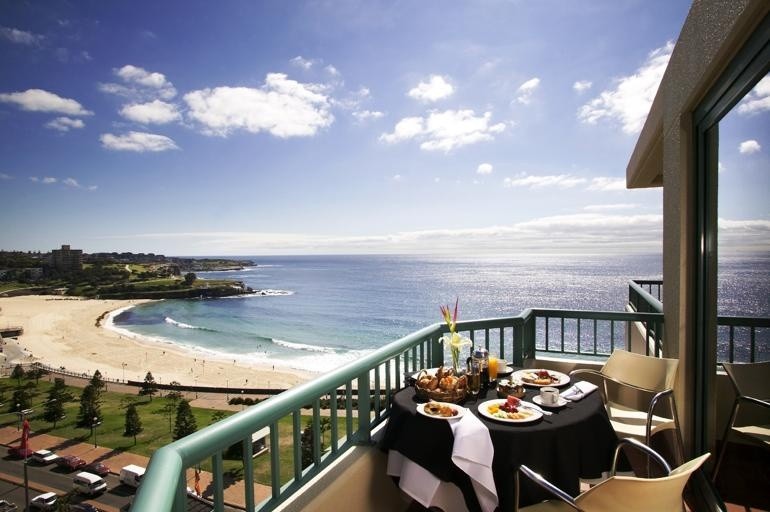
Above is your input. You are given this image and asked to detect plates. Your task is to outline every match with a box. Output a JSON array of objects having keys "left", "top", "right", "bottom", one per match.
[
  {"left": 532, "top": 394, "right": 568, "bottom": 408},
  {"left": 497, "top": 367, "right": 514, "bottom": 375},
  {"left": 511, "top": 368, "right": 571, "bottom": 387},
  {"left": 416, "top": 402, "right": 465, "bottom": 420},
  {"left": 478, "top": 398, "right": 544, "bottom": 423}
]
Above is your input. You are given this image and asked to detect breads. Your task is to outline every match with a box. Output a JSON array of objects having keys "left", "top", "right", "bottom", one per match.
[{"left": 419, "top": 374, "right": 468, "bottom": 390}]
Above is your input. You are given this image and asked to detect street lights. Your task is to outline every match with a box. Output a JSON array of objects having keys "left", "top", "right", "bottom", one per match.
[
  {"left": 16, "top": 402, "right": 21, "bottom": 431},
  {"left": 0, "top": 398, "right": 58, "bottom": 511},
  {"left": 241, "top": 389, "right": 245, "bottom": 410},
  {"left": 92, "top": 416, "right": 102, "bottom": 448}
]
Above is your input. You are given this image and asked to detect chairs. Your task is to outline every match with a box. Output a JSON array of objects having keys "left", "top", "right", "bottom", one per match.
[
  {"left": 513, "top": 349, "right": 711, "bottom": 511},
  {"left": 714, "top": 358, "right": 770, "bottom": 483}
]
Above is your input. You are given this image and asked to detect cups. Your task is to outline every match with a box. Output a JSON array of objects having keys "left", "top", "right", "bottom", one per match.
[
  {"left": 498, "top": 360, "right": 508, "bottom": 371},
  {"left": 487, "top": 352, "right": 498, "bottom": 383},
  {"left": 540, "top": 387, "right": 559, "bottom": 404}
]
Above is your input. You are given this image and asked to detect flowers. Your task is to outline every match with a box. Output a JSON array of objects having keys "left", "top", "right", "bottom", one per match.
[{"left": 438, "top": 296, "right": 472, "bottom": 373}]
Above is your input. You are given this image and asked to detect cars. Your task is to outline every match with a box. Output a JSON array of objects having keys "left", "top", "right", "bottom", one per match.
[{"left": 0, "top": 444, "right": 148, "bottom": 512}]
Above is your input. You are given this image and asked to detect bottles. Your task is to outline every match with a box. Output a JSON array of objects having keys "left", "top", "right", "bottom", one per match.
[
  {"left": 465, "top": 356, "right": 480, "bottom": 396},
  {"left": 472, "top": 344, "right": 488, "bottom": 390}
]
